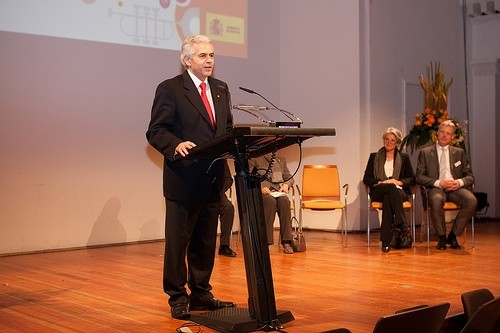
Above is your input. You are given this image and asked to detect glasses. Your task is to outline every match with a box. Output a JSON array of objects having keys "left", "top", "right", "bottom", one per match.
[{"left": 383, "top": 139, "right": 396, "bottom": 144}]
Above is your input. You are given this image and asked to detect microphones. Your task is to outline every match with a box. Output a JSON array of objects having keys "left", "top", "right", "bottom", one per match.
[{"left": 239, "top": 87, "right": 294, "bottom": 121}]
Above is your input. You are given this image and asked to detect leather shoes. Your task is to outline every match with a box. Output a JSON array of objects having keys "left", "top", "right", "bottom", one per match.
[
  {"left": 171, "top": 304, "right": 191, "bottom": 320},
  {"left": 190, "top": 292, "right": 237, "bottom": 310}
]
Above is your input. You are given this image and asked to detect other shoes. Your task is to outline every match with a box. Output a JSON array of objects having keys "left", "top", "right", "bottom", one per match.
[
  {"left": 284, "top": 244, "right": 293, "bottom": 254},
  {"left": 447, "top": 236, "right": 462, "bottom": 249},
  {"left": 382, "top": 242, "right": 390, "bottom": 252},
  {"left": 437, "top": 238, "right": 447, "bottom": 250},
  {"left": 219, "top": 246, "right": 237, "bottom": 256}
]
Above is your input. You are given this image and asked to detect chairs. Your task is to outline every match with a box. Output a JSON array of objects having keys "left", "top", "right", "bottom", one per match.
[
  {"left": 421, "top": 184, "right": 475, "bottom": 248},
  {"left": 321, "top": 288, "right": 500, "bottom": 333},
  {"left": 298, "top": 165, "right": 349, "bottom": 248},
  {"left": 237, "top": 185, "right": 297, "bottom": 248},
  {"left": 367, "top": 186, "right": 417, "bottom": 247}
]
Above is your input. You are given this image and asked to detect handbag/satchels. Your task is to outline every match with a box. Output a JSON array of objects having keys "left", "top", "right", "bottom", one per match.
[
  {"left": 291, "top": 216, "right": 307, "bottom": 252},
  {"left": 392, "top": 224, "right": 412, "bottom": 248}
]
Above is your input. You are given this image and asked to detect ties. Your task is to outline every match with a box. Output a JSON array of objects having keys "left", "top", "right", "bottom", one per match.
[
  {"left": 439, "top": 148, "right": 447, "bottom": 181},
  {"left": 199, "top": 83, "right": 215, "bottom": 127}
]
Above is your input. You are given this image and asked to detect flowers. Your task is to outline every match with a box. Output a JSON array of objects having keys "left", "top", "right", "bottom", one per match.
[{"left": 399, "top": 60, "right": 468, "bottom": 155}]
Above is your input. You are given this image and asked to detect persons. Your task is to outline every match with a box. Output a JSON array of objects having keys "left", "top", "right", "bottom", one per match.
[
  {"left": 362, "top": 127, "right": 416, "bottom": 252},
  {"left": 416, "top": 120, "right": 477, "bottom": 251},
  {"left": 145, "top": 34, "right": 237, "bottom": 319},
  {"left": 218, "top": 161, "right": 236, "bottom": 257},
  {"left": 248, "top": 153, "right": 294, "bottom": 254}
]
